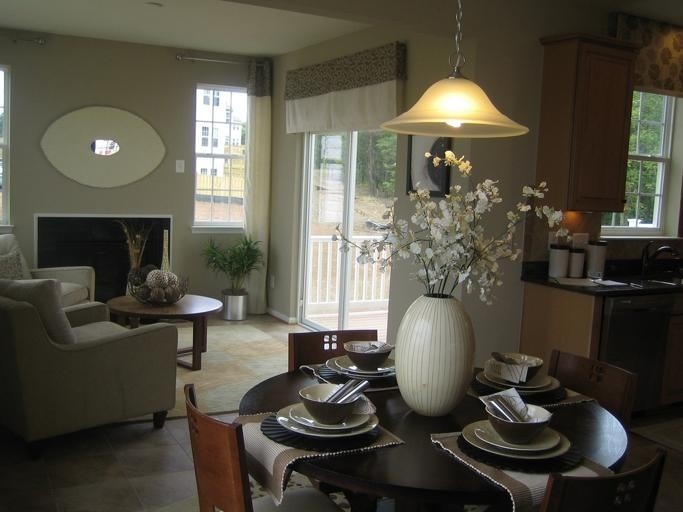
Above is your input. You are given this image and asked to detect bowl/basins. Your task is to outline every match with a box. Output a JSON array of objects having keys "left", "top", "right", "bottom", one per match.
[
  {"left": 298, "top": 383, "right": 361, "bottom": 426},
  {"left": 343, "top": 340, "right": 391, "bottom": 371},
  {"left": 485, "top": 403, "right": 553, "bottom": 445},
  {"left": 489, "top": 353, "right": 544, "bottom": 385}
]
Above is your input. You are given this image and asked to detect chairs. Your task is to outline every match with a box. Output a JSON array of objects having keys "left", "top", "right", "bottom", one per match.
[
  {"left": 548, "top": 348, "right": 636, "bottom": 426},
  {"left": 540, "top": 448, "right": 664, "bottom": 512},
  {"left": 1, "top": 233, "right": 108, "bottom": 323},
  {"left": 288, "top": 330, "right": 379, "bottom": 372},
  {"left": 183, "top": 383, "right": 347, "bottom": 512},
  {"left": 2, "top": 278, "right": 178, "bottom": 457}
]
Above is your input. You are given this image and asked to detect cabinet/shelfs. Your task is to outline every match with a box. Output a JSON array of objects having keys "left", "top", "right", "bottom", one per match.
[
  {"left": 661, "top": 293, "right": 683, "bottom": 407},
  {"left": 535, "top": 30, "right": 643, "bottom": 212}
]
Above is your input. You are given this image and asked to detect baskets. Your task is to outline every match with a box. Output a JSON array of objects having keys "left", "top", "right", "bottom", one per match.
[{"left": 127, "top": 272, "right": 188, "bottom": 306}]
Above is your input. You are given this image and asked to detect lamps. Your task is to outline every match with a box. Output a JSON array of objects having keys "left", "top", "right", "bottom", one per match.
[{"left": 380, "top": 1, "right": 531, "bottom": 140}]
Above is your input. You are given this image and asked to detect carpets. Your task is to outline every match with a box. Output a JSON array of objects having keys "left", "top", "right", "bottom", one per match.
[{"left": 103, "top": 325, "right": 291, "bottom": 426}]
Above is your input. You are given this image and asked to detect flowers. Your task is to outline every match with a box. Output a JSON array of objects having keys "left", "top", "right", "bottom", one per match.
[{"left": 331, "top": 150, "right": 573, "bottom": 307}]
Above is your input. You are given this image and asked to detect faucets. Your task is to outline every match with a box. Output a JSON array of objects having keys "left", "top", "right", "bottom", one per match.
[{"left": 640, "top": 240, "right": 681, "bottom": 277}]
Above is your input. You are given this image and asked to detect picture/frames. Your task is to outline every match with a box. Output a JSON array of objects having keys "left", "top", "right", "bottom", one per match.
[{"left": 405, "top": 134, "right": 451, "bottom": 199}]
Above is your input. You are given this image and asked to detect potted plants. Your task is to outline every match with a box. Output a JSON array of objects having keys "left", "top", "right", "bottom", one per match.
[{"left": 200, "top": 234, "right": 264, "bottom": 321}]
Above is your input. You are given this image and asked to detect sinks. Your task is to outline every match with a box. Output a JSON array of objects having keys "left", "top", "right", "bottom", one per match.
[{"left": 621, "top": 276, "right": 683, "bottom": 288}]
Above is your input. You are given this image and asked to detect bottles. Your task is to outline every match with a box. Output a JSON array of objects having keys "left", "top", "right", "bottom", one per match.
[{"left": 548, "top": 243, "right": 570, "bottom": 278}]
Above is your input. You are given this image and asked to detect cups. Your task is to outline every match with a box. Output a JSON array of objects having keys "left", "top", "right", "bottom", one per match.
[
  {"left": 584, "top": 240, "right": 607, "bottom": 279},
  {"left": 568, "top": 248, "right": 585, "bottom": 278}
]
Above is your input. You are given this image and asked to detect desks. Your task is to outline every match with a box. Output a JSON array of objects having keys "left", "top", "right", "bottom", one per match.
[{"left": 240, "top": 358, "right": 627, "bottom": 512}]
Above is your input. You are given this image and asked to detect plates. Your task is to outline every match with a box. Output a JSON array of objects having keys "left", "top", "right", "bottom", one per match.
[
  {"left": 461, "top": 419, "right": 571, "bottom": 463},
  {"left": 326, "top": 354, "right": 396, "bottom": 381},
  {"left": 276, "top": 402, "right": 379, "bottom": 441},
  {"left": 475, "top": 370, "right": 561, "bottom": 396}
]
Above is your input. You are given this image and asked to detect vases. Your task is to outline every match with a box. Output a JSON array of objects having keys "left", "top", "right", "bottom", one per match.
[{"left": 393, "top": 292, "right": 476, "bottom": 418}]
